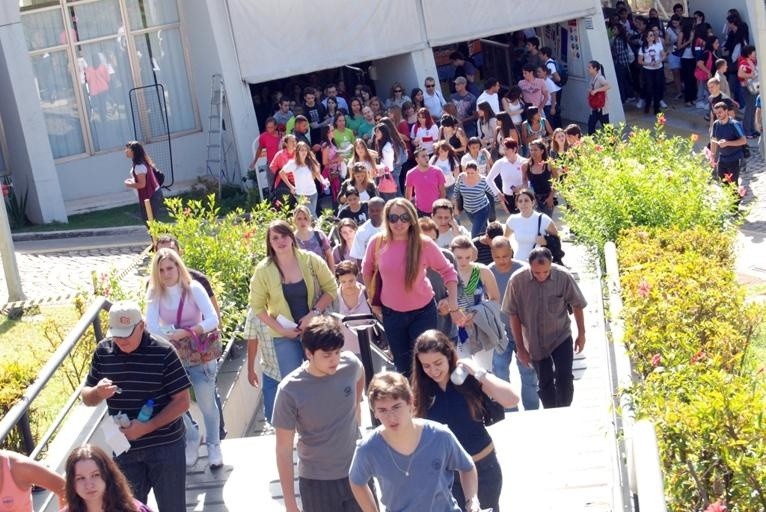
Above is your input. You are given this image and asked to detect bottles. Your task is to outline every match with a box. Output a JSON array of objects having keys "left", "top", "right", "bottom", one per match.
[{"left": 137, "top": 401, "right": 153, "bottom": 422}]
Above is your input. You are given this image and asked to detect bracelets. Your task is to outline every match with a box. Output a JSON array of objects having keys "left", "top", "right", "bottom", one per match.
[
  {"left": 449, "top": 308, "right": 460, "bottom": 313},
  {"left": 474, "top": 367, "right": 487, "bottom": 379}
]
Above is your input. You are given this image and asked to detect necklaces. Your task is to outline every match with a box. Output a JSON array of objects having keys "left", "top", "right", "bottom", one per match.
[{"left": 384, "top": 422, "right": 420, "bottom": 477}]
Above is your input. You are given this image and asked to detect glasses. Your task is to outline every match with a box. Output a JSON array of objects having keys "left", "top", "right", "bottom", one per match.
[
  {"left": 426, "top": 84, "right": 437, "bottom": 88},
  {"left": 647, "top": 33, "right": 656, "bottom": 36},
  {"left": 393, "top": 88, "right": 404, "bottom": 93},
  {"left": 387, "top": 212, "right": 414, "bottom": 227}
]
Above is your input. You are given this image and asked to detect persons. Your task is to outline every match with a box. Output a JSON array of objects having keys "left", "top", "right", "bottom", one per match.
[
  {"left": 390, "top": 106, "right": 410, "bottom": 194},
  {"left": 321, "top": 82, "right": 349, "bottom": 114},
  {"left": 564, "top": 124, "right": 583, "bottom": 146},
  {"left": 286, "top": 103, "right": 312, "bottom": 149},
  {"left": 502, "top": 85, "right": 525, "bottom": 140},
  {"left": 722, "top": 9, "right": 749, "bottom": 46},
  {"left": 677, "top": 17, "right": 697, "bottom": 106},
  {"left": 477, "top": 77, "right": 500, "bottom": 140},
  {"left": 472, "top": 221, "right": 504, "bottom": 266},
  {"left": 485, "top": 138, "right": 529, "bottom": 216},
  {"left": 452, "top": 162, "right": 497, "bottom": 240},
  {"left": 449, "top": 52, "right": 482, "bottom": 98},
  {"left": 123, "top": 141, "right": 164, "bottom": 245},
  {"left": 427, "top": 140, "right": 461, "bottom": 200},
  {"left": 411, "top": 87, "right": 425, "bottom": 114},
  {"left": 245, "top": 306, "right": 283, "bottom": 437},
  {"left": 330, "top": 260, "right": 375, "bottom": 364},
  {"left": 271, "top": 315, "right": 379, "bottom": 512},
  {"left": 520, "top": 37, "right": 543, "bottom": 79},
  {"left": 500, "top": 247, "right": 588, "bottom": 409},
  {"left": 437, "top": 113, "right": 467, "bottom": 161},
  {"left": 714, "top": 58, "right": 731, "bottom": 97},
  {"left": 80, "top": 301, "right": 192, "bottom": 512},
  {"left": 57, "top": 445, "right": 153, "bottom": 512},
  {"left": 711, "top": 101, "right": 746, "bottom": 220},
  {"left": 144, "top": 248, "right": 223, "bottom": 470},
  {"left": 449, "top": 236, "right": 500, "bottom": 373},
  {"left": 269, "top": 134, "right": 298, "bottom": 218},
  {"left": 504, "top": 188, "right": 558, "bottom": 267},
  {"left": 361, "top": 197, "right": 468, "bottom": 391},
  {"left": 485, "top": 235, "right": 539, "bottom": 413},
  {"left": 536, "top": 64, "right": 562, "bottom": 131},
  {"left": 368, "top": 96, "right": 389, "bottom": 122},
  {"left": 358, "top": 105, "right": 378, "bottom": 151},
  {"left": 336, "top": 164, "right": 376, "bottom": 216},
  {"left": 549, "top": 127, "right": 570, "bottom": 210},
  {"left": 493, "top": 111, "right": 518, "bottom": 159},
  {"left": 332, "top": 113, "right": 356, "bottom": 166},
  {"left": 450, "top": 77, "right": 478, "bottom": 138},
  {"left": 272, "top": 97, "right": 294, "bottom": 132},
  {"left": 404, "top": 147, "right": 445, "bottom": 218},
  {"left": 248, "top": 116, "right": 285, "bottom": 199},
  {"left": 694, "top": 23, "right": 712, "bottom": 111},
  {"left": 320, "top": 124, "right": 343, "bottom": 217},
  {"left": 147, "top": 235, "right": 227, "bottom": 445},
  {"left": 410, "top": 107, "right": 439, "bottom": 160},
  {"left": 386, "top": 84, "right": 412, "bottom": 109},
  {"left": 292, "top": 205, "right": 335, "bottom": 315},
  {"left": 439, "top": 103, "right": 465, "bottom": 131},
  {"left": 607, "top": 0, "right": 668, "bottom": 117},
  {"left": 521, "top": 106, "right": 554, "bottom": 158},
  {"left": 476, "top": 101, "right": 499, "bottom": 163},
  {"left": 737, "top": 45, "right": 761, "bottom": 138},
  {"left": 707, "top": 77, "right": 736, "bottom": 164},
  {"left": 665, "top": 14, "right": 684, "bottom": 100},
  {"left": 323, "top": 96, "right": 342, "bottom": 133},
  {"left": 278, "top": 141, "right": 330, "bottom": 218},
  {"left": 295, "top": 87, "right": 326, "bottom": 173},
  {"left": 428, "top": 197, "right": 470, "bottom": 249},
  {"left": 721, "top": 15, "right": 745, "bottom": 113},
  {"left": 379, "top": 117, "right": 407, "bottom": 198},
  {"left": 668, "top": 3, "right": 685, "bottom": 28},
  {"left": 373, "top": 123, "right": 395, "bottom": 203},
  {"left": 422, "top": 77, "right": 447, "bottom": 128},
  {"left": 397, "top": 101, "right": 418, "bottom": 173},
  {"left": 512, "top": 141, "right": 558, "bottom": 220},
  {"left": 291, "top": 115, "right": 322, "bottom": 217},
  {"left": 360, "top": 85, "right": 386, "bottom": 122},
  {"left": 696, "top": 35, "right": 719, "bottom": 120},
  {"left": 348, "top": 371, "right": 483, "bottom": 512},
  {"left": 538, "top": 46, "right": 561, "bottom": 128},
  {"left": 408, "top": 330, "right": 521, "bottom": 512},
  {"left": 517, "top": 63, "right": 548, "bottom": 119},
  {"left": 0, "top": 449, "right": 68, "bottom": 512},
  {"left": 343, "top": 97, "right": 365, "bottom": 135},
  {"left": 342, "top": 138, "right": 385, "bottom": 197},
  {"left": 586, "top": 60, "right": 615, "bottom": 145},
  {"left": 248, "top": 220, "right": 338, "bottom": 383},
  {"left": 332, "top": 217, "right": 367, "bottom": 306},
  {"left": 338, "top": 187, "right": 370, "bottom": 228},
  {"left": 694, "top": 11, "right": 705, "bottom": 30}
]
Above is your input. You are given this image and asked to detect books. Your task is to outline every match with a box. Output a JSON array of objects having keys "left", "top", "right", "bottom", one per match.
[{"left": 34, "top": 15, "right": 153, "bottom": 124}]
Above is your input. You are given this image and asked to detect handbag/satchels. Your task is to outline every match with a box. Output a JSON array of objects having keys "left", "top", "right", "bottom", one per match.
[
  {"left": 169, "top": 278, "right": 223, "bottom": 368},
  {"left": 490, "top": 140, "right": 501, "bottom": 161},
  {"left": 587, "top": 74, "right": 607, "bottom": 109},
  {"left": 693, "top": 50, "right": 713, "bottom": 84},
  {"left": 464, "top": 373, "right": 509, "bottom": 429},
  {"left": 741, "top": 66, "right": 761, "bottom": 95},
  {"left": 377, "top": 170, "right": 397, "bottom": 195},
  {"left": 366, "top": 232, "right": 383, "bottom": 308},
  {"left": 453, "top": 177, "right": 464, "bottom": 214},
  {"left": 671, "top": 45, "right": 687, "bottom": 56},
  {"left": 150, "top": 163, "right": 165, "bottom": 187}
]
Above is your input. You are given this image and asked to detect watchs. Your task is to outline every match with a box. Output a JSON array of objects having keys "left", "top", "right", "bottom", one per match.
[{"left": 311, "top": 308, "right": 321, "bottom": 316}]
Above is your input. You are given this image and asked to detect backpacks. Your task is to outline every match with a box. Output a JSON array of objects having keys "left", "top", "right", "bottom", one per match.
[
  {"left": 715, "top": 120, "right": 751, "bottom": 158},
  {"left": 546, "top": 59, "right": 569, "bottom": 87}
]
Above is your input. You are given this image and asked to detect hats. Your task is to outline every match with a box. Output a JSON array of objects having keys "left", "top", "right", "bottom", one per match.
[
  {"left": 452, "top": 75, "right": 467, "bottom": 84},
  {"left": 103, "top": 299, "right": 144, "bottom": 341}
]
